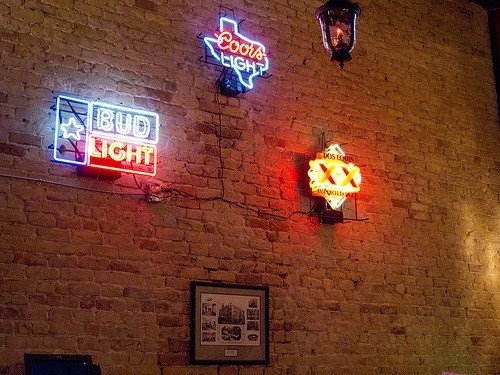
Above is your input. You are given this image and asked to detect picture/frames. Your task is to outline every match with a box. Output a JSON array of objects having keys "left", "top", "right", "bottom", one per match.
[{"left": 187, "top": 280, "right": 271, "bottom": 366}]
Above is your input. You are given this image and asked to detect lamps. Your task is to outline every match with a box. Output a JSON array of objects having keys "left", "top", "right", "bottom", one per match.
[
  {"left": 307, "top": 130, "right": 368, "bottom": 224},
  {"left": 312, "top": 0, "right": 364, "bottom": 70},
  {"left": 192, "top": 13, "right": 273, "bottom": 97},
  {"left": 47, "top": 94, "right": 161, "bottom": 182}
]
